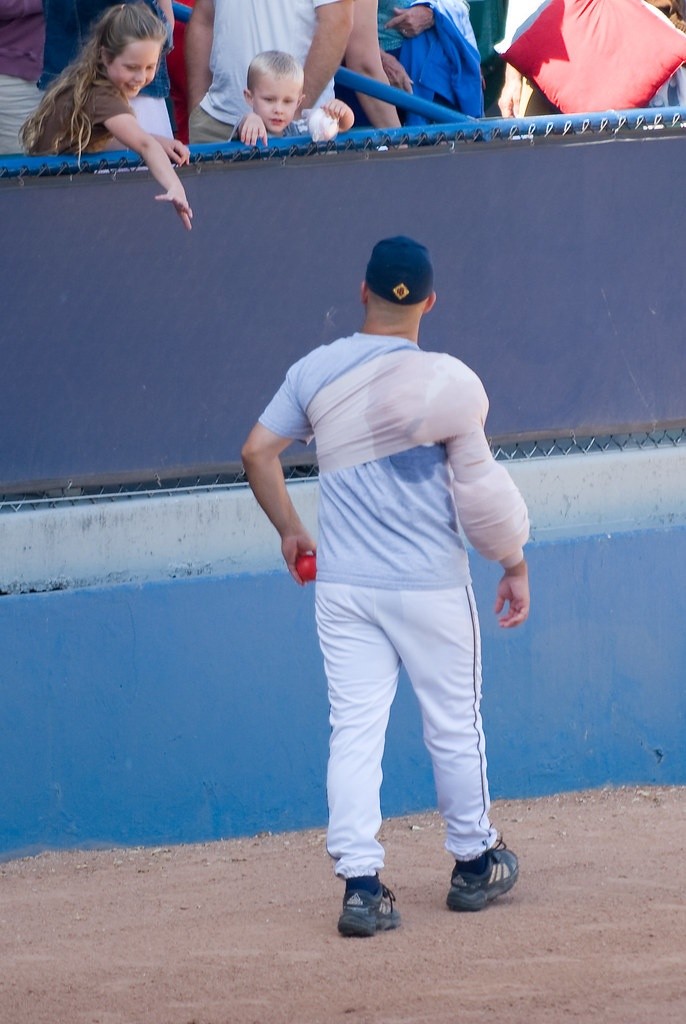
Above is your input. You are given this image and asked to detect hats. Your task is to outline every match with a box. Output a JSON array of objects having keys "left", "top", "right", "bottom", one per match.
[{"left": 365, "top": 235, "right": 434, "bottom": 305}]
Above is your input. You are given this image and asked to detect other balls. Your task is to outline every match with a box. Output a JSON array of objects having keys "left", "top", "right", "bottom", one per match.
[{"left": 293, "top": 550, "right": 320, "bottom": 585}]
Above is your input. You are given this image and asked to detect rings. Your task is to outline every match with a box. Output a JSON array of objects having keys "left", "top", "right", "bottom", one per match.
[{"left": 401, "top": 29, "right": 407, "bottom": 34}]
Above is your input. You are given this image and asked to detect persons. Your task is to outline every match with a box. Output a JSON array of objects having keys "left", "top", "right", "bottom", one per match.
[
  {"left": 238, "top": 50, "right": 355, "bottom": 147},
  {"left": 645, "top": 0, "right": 686, "bottom": 108},
  {"left": 240, "top": 236, "right": 529, "bottom": 940},
  {"left": 18, "top": 6, "right": 193, "bottom": 230},
  {"left": 187, "top": 0, "right": 354, "bottom": 145},
  {"left": 333, "top": 1, "right": 561, "bottom": 130},
  {"left": 0, "top": 0, "right": 174, "bottom": 155}
]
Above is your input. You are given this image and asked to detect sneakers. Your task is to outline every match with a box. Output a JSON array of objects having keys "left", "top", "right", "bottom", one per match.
[
  {"left": 446, "top": 833, "right": 520, "bottom": 912},
  {"left": 338, "top": 883, "right": 402, "bottom": 937}
]
